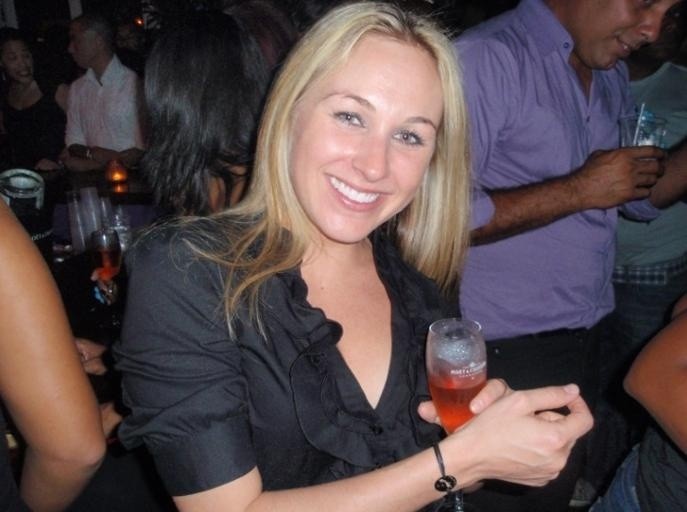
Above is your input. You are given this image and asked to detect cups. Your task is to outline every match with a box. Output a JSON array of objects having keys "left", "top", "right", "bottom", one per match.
[
  {"left": 622, "top": 115, "right": 665, "bottom": 153},
  {"left": 67, "top": 188, "right": 131, "bottom": 252}
]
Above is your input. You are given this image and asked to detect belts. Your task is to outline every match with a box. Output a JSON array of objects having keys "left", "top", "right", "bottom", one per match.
[{"left": 613, "top": 256, "right": 687, "bottom": 285}]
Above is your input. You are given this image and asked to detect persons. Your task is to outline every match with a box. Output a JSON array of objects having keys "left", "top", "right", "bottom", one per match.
[
  {"left": 109, "top": 0, "right": 595, "bottom": 512},
  {"left": 450, "top": 0, "right": 687, "bottom": 512},
  {"left": 0, "top": 13, "right": 148, "bottom": 172},
  {"left": 0, "top": 197, "right": 108, "bottom": 512},
  {"left": 75, "top": 1, "right": 299, "bottom": 437}
]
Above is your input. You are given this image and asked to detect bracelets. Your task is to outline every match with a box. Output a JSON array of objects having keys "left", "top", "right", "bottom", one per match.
[{"left": 432, "top": 442, "right": 457, "bottom": 495}]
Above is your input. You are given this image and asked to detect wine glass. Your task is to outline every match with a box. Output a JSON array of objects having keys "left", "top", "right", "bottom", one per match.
[
  {"left": 91, "top": 230, "right": 123, "bottom": 325},
  {"left": 425, "top": 318, "right": 489, "bottom": 512}
]
